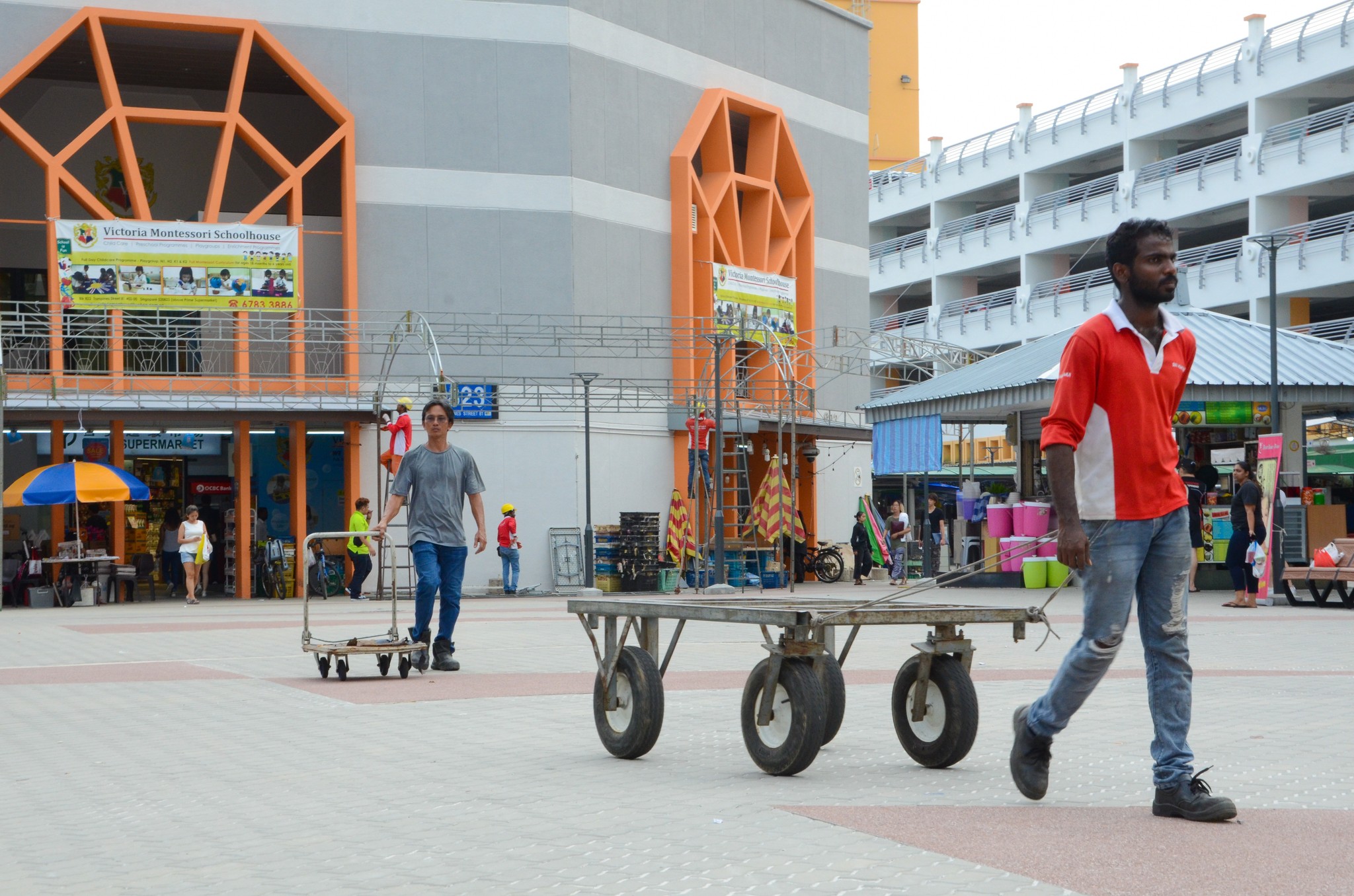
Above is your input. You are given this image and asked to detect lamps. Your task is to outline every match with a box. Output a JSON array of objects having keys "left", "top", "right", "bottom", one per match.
[
  {"left": 92, "top": 428, "right": 161, "bottom": 433},
  {"left": 17, "top": 427, "right": 88, "bottom": 433},
  {"left": 306, "top": 430, "right": 344, "bottom": 434},
  {"left": 164, "top": 430, "right": 233, "bottom": 434},
  {"left": 248, "top": 429, "right": 276, "bottom": 434}
]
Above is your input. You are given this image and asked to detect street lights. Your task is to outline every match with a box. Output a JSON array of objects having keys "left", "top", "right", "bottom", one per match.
[
  {"left": 1245, "top": 235, "right": 1304, "bottom": 595},
  {"left": 569, "top": 371, "right": 605, "bottom": 589}
]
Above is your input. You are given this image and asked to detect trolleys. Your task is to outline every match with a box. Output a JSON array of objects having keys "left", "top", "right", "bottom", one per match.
[{"left": 301, "top": 530, "right": 428, "bottom": 681}]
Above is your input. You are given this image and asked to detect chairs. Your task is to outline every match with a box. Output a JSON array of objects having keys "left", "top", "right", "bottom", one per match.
[
  {"left": 124, "top": 552, "right": 155, "bottom": 601},
  {"left": 1280, "top": 538, "right": 1354, "bottom": 608}
]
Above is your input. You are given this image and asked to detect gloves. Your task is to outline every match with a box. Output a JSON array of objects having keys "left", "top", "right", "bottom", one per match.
[
  {"left": 382, "top": 413, "right": 391, "bottom": 423},
  {"left": 509, "top": 537, "right": 518, "bottom": 546},
  {"left": 516, "top": 541, "right": 522, "bottom": 549}
]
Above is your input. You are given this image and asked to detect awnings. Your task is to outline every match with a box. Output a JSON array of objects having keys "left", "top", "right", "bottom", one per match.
[{"left": 185, "top": 476, "right": 232, "bottom": 494}]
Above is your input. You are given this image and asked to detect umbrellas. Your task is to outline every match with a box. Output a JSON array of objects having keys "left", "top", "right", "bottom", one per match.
[
  {"left": 3, "top": 458, "right": 153, "bottom": 574},
  {"left": 665, "top": 489, "right": 705, "bottom": 582},
  {"left": 742, "top": 452, "right": 807, "bottom": 566},
  {"left": 858, "top": 494, "right": 894, "bottom": 575}
]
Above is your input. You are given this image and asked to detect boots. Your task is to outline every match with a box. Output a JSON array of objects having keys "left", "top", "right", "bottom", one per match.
[
  {"left": 431, "top": 639, "right": 460, "bottom": 670},
  {"left": 407, "top": 627, "right": 431, "bottom": 670}
]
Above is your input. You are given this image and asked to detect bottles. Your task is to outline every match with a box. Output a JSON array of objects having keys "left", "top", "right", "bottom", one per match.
[
  {"left": 1313, "top": 489, "right": 1325, "bottom": 505},
  {"left": 1301, "top": 487, "right": 1313, "bottom": 505}
]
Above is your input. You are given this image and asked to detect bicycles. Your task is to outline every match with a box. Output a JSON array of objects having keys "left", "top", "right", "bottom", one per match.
[
  {"left": 783, "top": 533, "right": 844, "bottom": 584},
  {"left": 307, "top": 537, "right": 341, "bottom": 600},
  {"left": 253, "top": 534, "right": 287, "bottom": 599}
]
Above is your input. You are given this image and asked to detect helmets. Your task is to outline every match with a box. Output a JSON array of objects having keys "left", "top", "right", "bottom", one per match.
[
  {"left": 397, "top": 397, "right": 412, "bottom": 410},
  {"left": 501, "top": 503, "right": 517, "bottom": 514}
]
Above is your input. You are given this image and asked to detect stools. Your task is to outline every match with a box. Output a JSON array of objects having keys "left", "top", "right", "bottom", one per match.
[{"left": 107, "top": 574, "right": 137, "bottom": 602}]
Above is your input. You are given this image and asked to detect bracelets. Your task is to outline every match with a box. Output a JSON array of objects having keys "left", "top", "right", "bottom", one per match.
[
  {"left": 882, "top": 537, "right": 886, "bottom": 539},
  {"left": 366, "top": 516, "right": 371, "bottom": 520},
  {"left": 897, "top": 532, "right": 900, "bottom": 536}
]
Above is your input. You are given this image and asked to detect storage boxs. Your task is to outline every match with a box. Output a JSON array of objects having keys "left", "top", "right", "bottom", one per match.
[
  {"left": 274, "top": 535, "right": 296, "bottom": 599},
  {"left": 908, "top": 540, "right": 941, "bottom": 579},
  {"left": 109, "top": 511, "right": 147, "bottom": 565},
  {"left": 28, "top": 586, "right": 54, "bottom": 608},
  {"left": 594, "top": 510, "right": 679, "bottom": 591},
  {"left": 686, "top": 536, "right": 788, "bottom": 589}
]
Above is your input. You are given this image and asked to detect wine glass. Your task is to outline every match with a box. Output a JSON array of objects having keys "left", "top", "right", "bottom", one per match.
[{"left": 1203, "top": 512, "right": 1210, "bottom": 520}]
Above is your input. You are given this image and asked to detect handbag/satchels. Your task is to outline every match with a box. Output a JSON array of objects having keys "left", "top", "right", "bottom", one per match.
[
  {"left": 203, "top": 534, "right": 210, "bottom": 561},
  {"left": 890, "top": 521, "right": 904, "bottom": 538},
  {"left": 1245, "top": 540, "right": 1267, "bottom": 578},
  {"left": 497, "top": 546, "right": 501, "bottom": 557},
  {"left": 1309, "top": 542, "right": 1344, "bottom": 567},
  {"left": 195, "top": 534, "right": 207, "bottom": 564},
  {"left": 932, "top": 533, "right": 949, "bottom": 545}
]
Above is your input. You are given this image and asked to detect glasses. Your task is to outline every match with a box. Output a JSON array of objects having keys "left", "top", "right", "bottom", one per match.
[{"left": 424, "top": 415, "right": 449, "bottom": 423}]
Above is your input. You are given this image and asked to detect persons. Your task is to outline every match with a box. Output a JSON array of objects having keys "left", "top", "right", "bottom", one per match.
[
  {"left": 306, "top": 505, "right": 315, "bottom": 530},
  {"left": 763, "top": 309, "right": 772, "bottom": 327},
  {"left": 273, "top": 475, "right": 290, "bottom": 494},
  {"left": 71, "top": 265, "right": 117, "bottom": 294},
  {"left": 257, "top": 269, "right": 288, "bottom": 296},
  {"left": 176, "top": 503, "right": 211, "bottom": 603},
  {"left": 128, "top": 266, "right": 147, "bottom": 284},
  {"left": 782, "top": 312, "right": 794, "bottom": 334},
  {"left": 1187, "top": 445, "right": 1219, "bottom": 490},
  {"left": 344, "top": 498, "right": 376, "bottom": 601},
  {"left": 684, "top": 400, "right": 724, "bottom": 499},
  {"left": 379, "top": 397, "right": 413, "bottom": 506},
  {"left": 751, "top": 305, "right": 759, "bottom": 325},
  {"left": 881, "top": 500, "right": 911, "bottom": 585},
  {"left": 220, "top": 268, "right": 233, "bottom": 290},
  {"left": 175, "top": 267, "right": 198, "bottom": 295},
  {"left": 156, "top": 494, "right": 232, "bottom": 598},
  {"left": 85, "top": 503, "right": 111, "bottom": 536},
  {"left": 735, "top": 304, "right": 741, "bottom": 319},
  {"left": 364, "top": 400, "right": 488, "bottom": 668},
  {"left": 256, "top": 507, "right": 270, "bottom": 540},
  {"left": 497, "top": 503, "right": 522, "bottom": 595},
  {"left": 1010, "top": 218, "right": 1238, "bottom": 822},
  {"left": 717, "top": 303, "right": 734, "bottom": 325},
  {"left": 851, "top": 512, "right": 873, "bottom": 586},
  {"left": 26, "top": 521, "right": 50, "bottom": 551},
  {"left": 784, "top": 508, "right": 807, "bottom": 584},
  {"left": 918, "top": 492, "right": 946, "bottom": 578},
  {"left": 1176, "top": 458, "right": 1208, "bottom": 592},
  {"left": 1222, "top": 460, "right": 1267, "bottom": 608}
]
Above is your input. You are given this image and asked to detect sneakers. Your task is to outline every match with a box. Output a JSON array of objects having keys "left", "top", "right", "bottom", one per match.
[
  {"left": 1152, "top": 765, "right": 1237, "bottom": 821},
  {"left": 1010, "top": 703, "right": 1053, "bottom": 800}
]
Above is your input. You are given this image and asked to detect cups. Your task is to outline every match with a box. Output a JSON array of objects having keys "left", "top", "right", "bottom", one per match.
[{"left": 1207, "top": 492, "right": 1218, "bottom": 505}]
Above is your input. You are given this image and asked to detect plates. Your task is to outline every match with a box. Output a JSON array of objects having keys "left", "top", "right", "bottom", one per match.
[{"left": 1204, "top": 523, "right": 1213, "bottom": 562}]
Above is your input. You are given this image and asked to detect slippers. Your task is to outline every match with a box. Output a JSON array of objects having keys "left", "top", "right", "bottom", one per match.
[
  {"left": 899, "top": 580, "right": 907, "bottom": 584},
  {"left": 890, "top": 582, "right": 898, "bottom": 585},
  {"left": 1221, "top": 602, "right": 1257, "bottom": 608},
  {"left": 854, "top": 578, "right": 866, "bottom": 585},
  {"left": 186, "top": 597, "right": 191, "bottom": 604},
  {"left": 190, "top": 599, "right": 199, "bottom": 604},
  {"left": 1188, "top": 586, "right": 1201, "bottom": 592}
]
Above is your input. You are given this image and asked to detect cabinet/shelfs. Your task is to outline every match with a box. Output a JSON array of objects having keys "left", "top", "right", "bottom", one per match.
[{"left": 224, "top": 509, "right": 256, "bottom": 594}]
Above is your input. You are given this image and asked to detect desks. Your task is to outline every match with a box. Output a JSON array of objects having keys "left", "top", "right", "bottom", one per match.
[{"left": 42, "top": 556, "right": 120, "bottom": 605}]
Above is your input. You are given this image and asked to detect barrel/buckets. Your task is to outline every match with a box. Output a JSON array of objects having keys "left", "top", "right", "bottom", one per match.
[
  {"left": 1008, "top": 534, "right": 1036, "bottom": 571},
  {"left": 1020, "top": 555, "right": 1047, "bottom": 588},
  {"left": 1046, "top": 554, "right": 1068, "bottom": 587},
  {"left": 1013, "top": 503, "right": 1024, "bottom": 536},
  {"left": 999, "top": 538, "right": 1012, "bottom": 572},
  {"left": 986, "top": 504, "right": 1013, "bottom": 537},
  {"left": 956, "top": 481, "right": 981, "bottom": 520},
  {"left": 1036, "top": 537, "right": 1058, "bottom": 557},
  {"left": 1019, "top": 501, "right": 1051, "bottom": 537}
]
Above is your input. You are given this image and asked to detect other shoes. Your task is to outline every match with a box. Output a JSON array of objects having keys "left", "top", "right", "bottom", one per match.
[
  {"left": 345, "top": 586, "right": 351, "bottom": 594},
  {"left": 201, "top": 591, "right": 207, "bottom": 600},
  {"left": 350, "top": 594, "right": 369, "bottom": 601},
  {"left": 194, "top": 585, "right": 202, "bottom": 596},
  {"left": 505, "top": 590, "right": 509, "bottom": 594},
  {"left": 165, "top": 582, "right": 174, "bottom": 595},
  {"left": 509, "top": 590, "right": 517, "bottom": 594},
  {"left": 171, "top": 592, "right": 177, "bottom": 597},
  {"left": 402, "top": 497, "right": 407, "bottom": 504}
]
